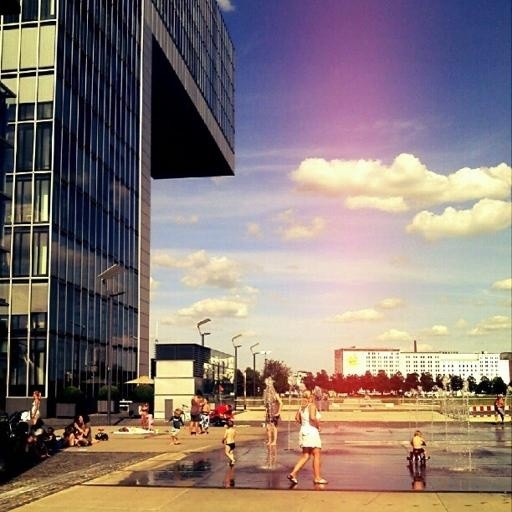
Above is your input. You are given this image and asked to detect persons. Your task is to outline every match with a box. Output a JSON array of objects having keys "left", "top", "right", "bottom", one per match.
[
  {"left": 214, "top": 405, "right": 233, "bottom": 426},
  {"left": 223, "top": 421, "right": 236, "bottom": 465},
  {"left": 264, "top": 390, "right": 282, "bottom": 446},
  {"left": 287, "top": 390, "right": 328, "bottom": 484},
  {"left": 28, "top": 391, "right": 91, "bottom": 456},
  {"left": 493, "top": 394, "right": 509, "bottom": 422},
  {"left": 94, "top": 428, "right": 108, "bottom": 441},
  {"left": 411, "top": 431, "right": 427, "bottom": 454},
  {"left": 168, "top": 392, "right": 210, "bottom": 445},
  {"left": 138, "top": 402, "right": 149, "bottom": 426}
]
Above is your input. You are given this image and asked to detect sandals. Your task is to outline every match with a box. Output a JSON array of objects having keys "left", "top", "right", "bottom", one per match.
[
  {"left": 287, "top": 475, "right": 297, "bottom": 483},
  {"left": 313, "top": 478, "right": 328, "bottom": 483}
]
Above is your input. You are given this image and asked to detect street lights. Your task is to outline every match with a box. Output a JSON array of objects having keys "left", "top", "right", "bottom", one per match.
[
  {"left": 231, "top": 334, "right": 244, "bottom": 405},
  {"left": 98, "top": 262, "right": 126, "bottom": 423},
  {"left": 251, "top": 342, "right": 270, "bottom": 397},
  {"left": 197, "top": 317, "right": 211, "bottom": 346}
]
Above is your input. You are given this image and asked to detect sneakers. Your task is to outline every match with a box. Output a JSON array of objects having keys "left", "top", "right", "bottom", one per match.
[{"left": 229, "top": 460, "right": 235, "bottom": 466}]
